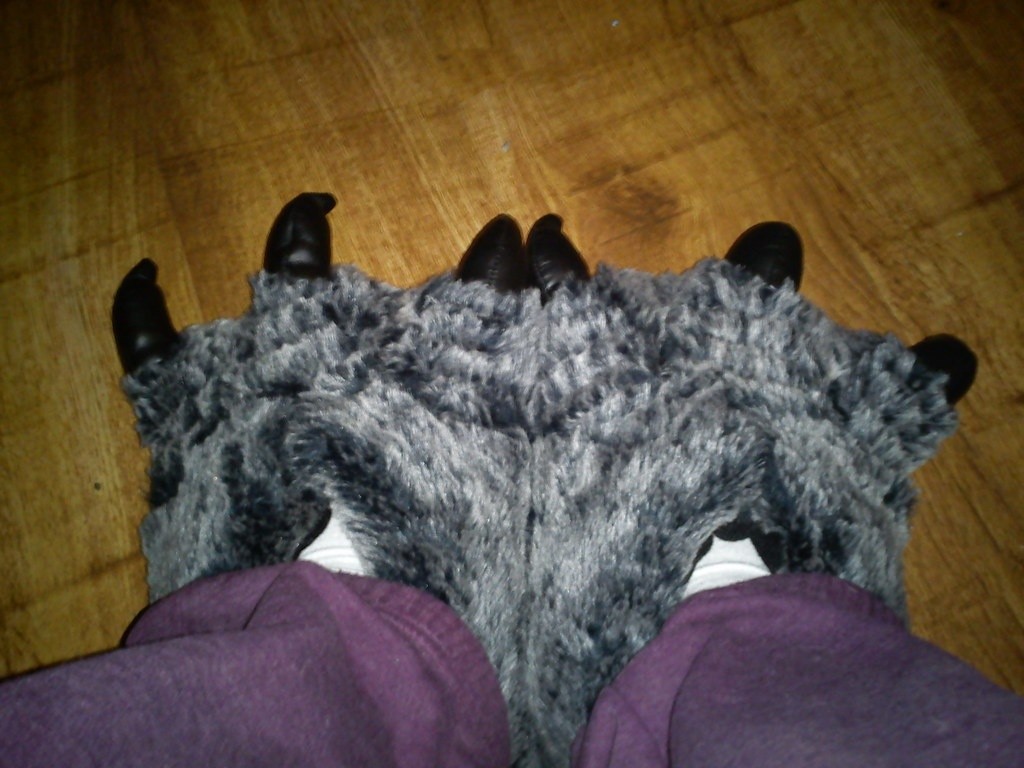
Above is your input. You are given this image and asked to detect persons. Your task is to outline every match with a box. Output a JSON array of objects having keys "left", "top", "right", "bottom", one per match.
[{"left": 0, "top": 193, "right": 1024, "bottom": 768}]
